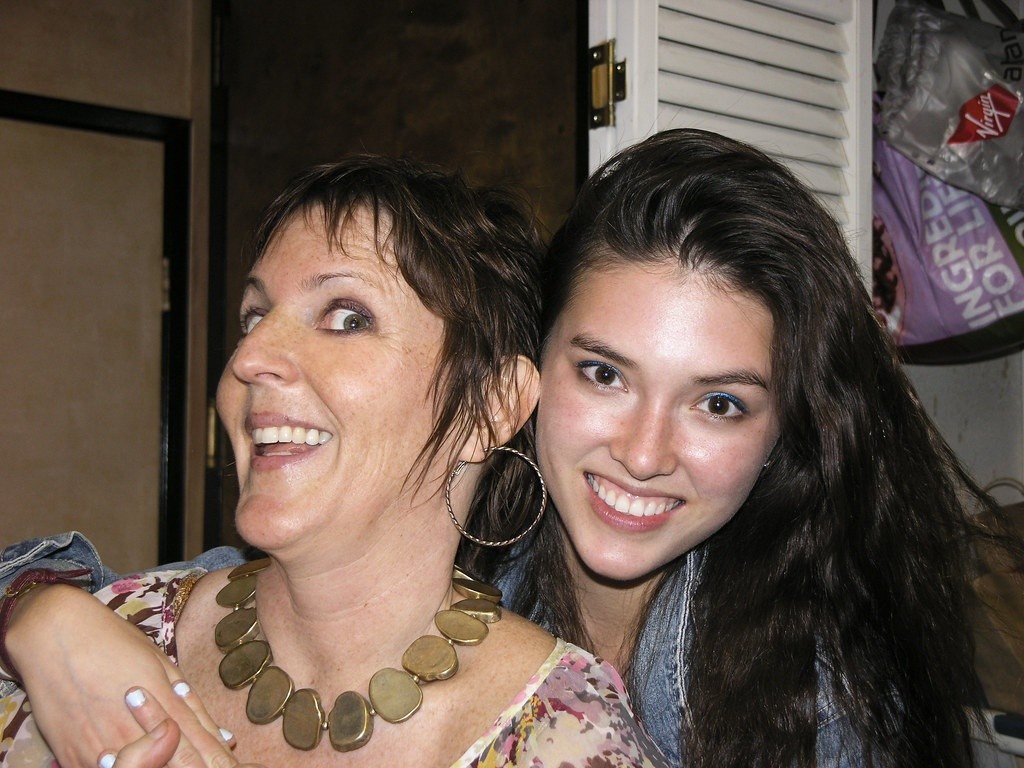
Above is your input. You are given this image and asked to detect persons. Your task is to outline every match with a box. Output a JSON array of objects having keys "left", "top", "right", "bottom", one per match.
[
  {"left": 0, "top": 150, "right": 679, "bottom": 768},
  {"left": 0, "top": 127, "right": 1024, "bottom": 768}
]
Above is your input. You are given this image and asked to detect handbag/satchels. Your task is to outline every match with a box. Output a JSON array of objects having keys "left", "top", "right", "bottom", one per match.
[
  {"left": 956, "top": 478, "right": 1024, "bottom": 718},
  {"left": 871, "top": 0, "right": 1024, "bottom": 347}
]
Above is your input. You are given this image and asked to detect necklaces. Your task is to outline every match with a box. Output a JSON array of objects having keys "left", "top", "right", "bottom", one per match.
[{"left": 214, "top": 558, "right": 504, "bottom": 753}]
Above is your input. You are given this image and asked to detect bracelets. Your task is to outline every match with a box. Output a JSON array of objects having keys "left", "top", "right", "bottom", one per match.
[{"left": 0, "top": 568, "right": 92, "bottom": 684}]
